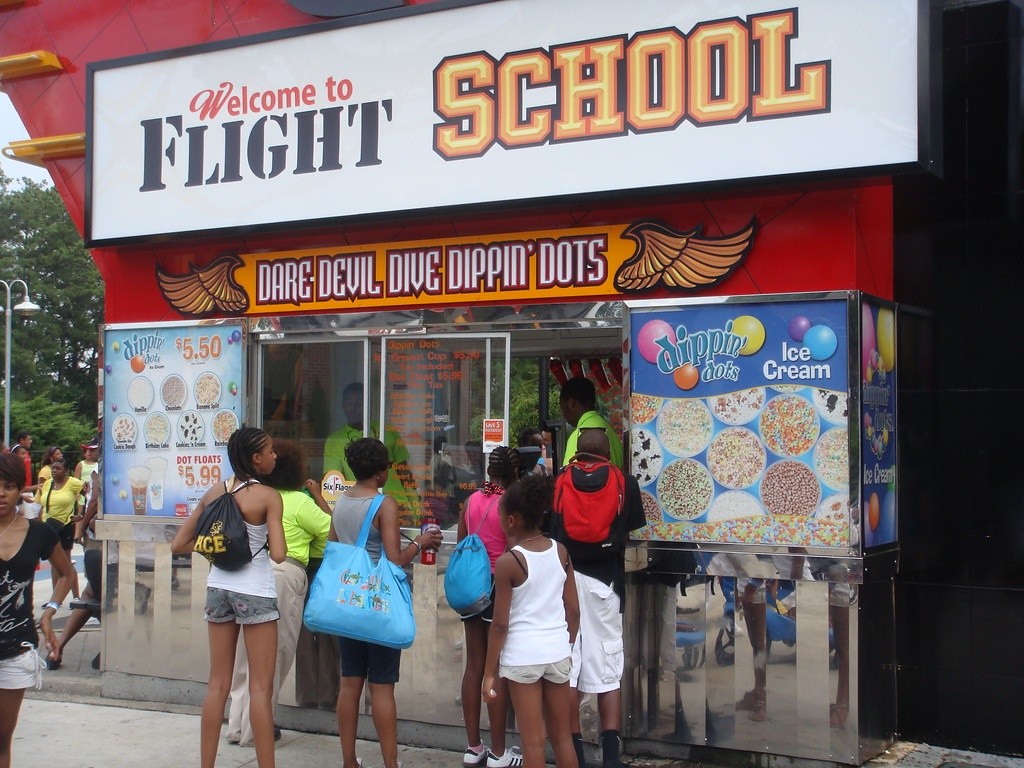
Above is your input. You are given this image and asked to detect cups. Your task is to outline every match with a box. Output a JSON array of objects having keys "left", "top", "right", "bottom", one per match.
[
  {"left": 145, "top": 456, "right": 168, "bottom": 510},
  {"left": 127, "top": 465, "right": 151, "bottom": 515}
]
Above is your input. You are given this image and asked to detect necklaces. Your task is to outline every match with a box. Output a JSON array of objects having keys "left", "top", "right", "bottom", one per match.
[
  {"left": 1, "top": 512, "right": 16, "bottom": 539},
  {"left": 519, "top": 534, "right": 544, "bottom": 542},
  {"left": 53, "top": 485, "right": 57, "bottom": 489}
]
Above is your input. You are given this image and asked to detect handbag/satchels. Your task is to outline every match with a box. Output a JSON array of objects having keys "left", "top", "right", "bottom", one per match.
[
  {"left": 303, "top": 540, "right": 416, "bottom": 650},
  {"left": 46, "top": 517, "right": 64, "bottom": 535}
]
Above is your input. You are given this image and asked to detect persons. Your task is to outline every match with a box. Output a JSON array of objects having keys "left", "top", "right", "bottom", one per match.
[
  {"left": 427, "top": 435, "right": 484, "bottom": 530},
  {"left": 549, "top": 430, "right": 647, "bottom": 768},
  {"left": 226, "top": 439, "right": 333, "bottom": 748},
  {"left": 518, "top": 427, "right": 552, "bottom": 476},
  {"left": 0, "top": 452, "right": 77, "bottom": 768},
  {"left": 457, "top": 446, "right": 524, "bottom": 768},
  {"left": 324, "top": 383, "right": 422, "bottom": 528},
  {"left": 172, "top": 429, "right": 288, "bottom": 768},
  {"left": 326, "top": 437, "right": 443, "bottom": 768},
  {"left": 666, "top": 547, "right": 850, "bottom": 746},
  {"left": 482, "top": 482, "right": 580, "bottom": 768},
  {"left": 0, "top": 430, "right": 102, "bottom": 669},
  {"left": 560, "top": 375, "right": 623, "bottom": 469}
]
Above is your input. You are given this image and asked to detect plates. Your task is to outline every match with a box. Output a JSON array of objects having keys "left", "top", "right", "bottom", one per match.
[
  {"left": 656, "top": 459, "right": 715, "bottom": 522},
  {"left": 760, "top": 459, "right": 823, "bottom": 518},
  {"left": 640, "top": 488, "right": 664, "bottom": 523},
  {"left": 706, "top": 426, "right": 767, "bottom": 490},
  {"left": 758, "top": 386, "right": 820, "bottom": 458},
  {"left": 815, "top": 491, "right": 849, "bottom": 523},
  {"left": 127, "top": 376, "right": 155, "bottom": 410},
  {"left": 707, "top": 490, "right": 767, "bottom": 523},
  {"left": 630, "top": 426, "right": 663, "bottom": 487},
  {"left": 813, "top": 426, "right": 849, "bottom": 492},
  {"left": 705, "top": 387, "right": 766, "bottom": 426},
  {"left": 160, "top": 374, "right": 187, "bottom": 407},
  {"left": 656, "top": 399, "right": 714, "bottom": 458},
  {"left": 177, "top": 409, "right": 205, "bottom": 444},
  {"left": 211, "top": 408, "right": 239, "bottom": 441},
  {"left": 810, "top": 386, "right": 848, "bottom": 426},
  {"left": 143, "top": 411, "right": 171, "bottom": 445},
  {"left": 193, "top": 372, "right": 222, "bottom": 404},
  {"left": 631, "top": 393, "right": 664, "bottom": 426},
  {"left": 111, "top": 414, "right": 138, "bottom": 445}
]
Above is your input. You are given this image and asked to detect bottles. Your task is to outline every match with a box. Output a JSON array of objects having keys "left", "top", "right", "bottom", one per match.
[{"left": 419, "top": 516, "right": 440, "bottom": 565}]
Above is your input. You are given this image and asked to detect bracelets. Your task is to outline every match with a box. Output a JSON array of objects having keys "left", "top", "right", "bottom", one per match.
[{"left": 46, "top": 600, "right": 59, "bottom": 613}]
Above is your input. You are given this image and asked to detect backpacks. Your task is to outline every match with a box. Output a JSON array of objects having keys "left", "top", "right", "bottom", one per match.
[
  {"left": 193, "top": 481, "right": 269, "bottom": 573},
  {"left": 552, "top": 452, "right": 632, "bottom": 557},
  {"left": 443, "top": 495, "right": 496, "bottom": 615}
]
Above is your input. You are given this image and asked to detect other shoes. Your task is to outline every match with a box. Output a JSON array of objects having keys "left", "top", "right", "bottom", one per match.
[
  {"left": 45, "top": 652, "right": 62, "bottom": 670},
  {"left": 274, "top": 728, "right": 282, "bottom": 741},
  {"left": 719, "top": 691, "right": 767, "bottom": 721},
  {"left": 830, "top": 703, "right": 849, "bottom": 728}
]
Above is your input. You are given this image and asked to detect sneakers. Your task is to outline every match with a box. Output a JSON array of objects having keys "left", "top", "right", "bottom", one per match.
[
  {"left": 486, "top": 745, "right": 523, "bottom": 768},
  {"left": 463, "top": 744, "right": 491, "bottom": 768}
]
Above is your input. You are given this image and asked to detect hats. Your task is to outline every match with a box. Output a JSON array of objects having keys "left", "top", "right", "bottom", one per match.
[{"left": 79, "top": 436, "right": 98, "bottom": 449}]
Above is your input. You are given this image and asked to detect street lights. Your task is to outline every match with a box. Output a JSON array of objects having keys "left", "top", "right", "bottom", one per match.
[{"left": 0, "top": 278, "right": 43, "bottom": 452}]
